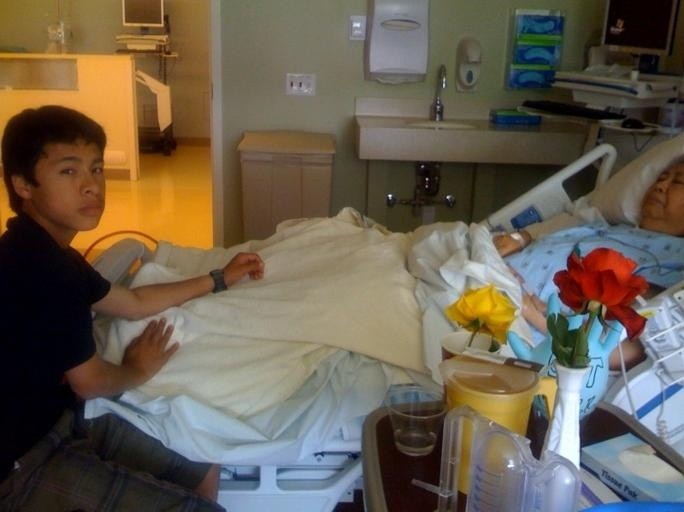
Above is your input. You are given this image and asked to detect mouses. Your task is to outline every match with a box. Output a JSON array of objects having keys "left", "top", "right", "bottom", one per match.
[{"left": 620, "top": 118, "right": 646, "bottom": 129}]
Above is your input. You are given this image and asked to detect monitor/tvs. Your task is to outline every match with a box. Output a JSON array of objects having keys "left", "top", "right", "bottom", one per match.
[{"left": 601, "top": 0, "right": 684, "bottom": 80}]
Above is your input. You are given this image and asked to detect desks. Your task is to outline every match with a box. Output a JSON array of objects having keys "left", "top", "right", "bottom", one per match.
[{"left": 358, "top": 395, "right": 684, "bottom": 512}]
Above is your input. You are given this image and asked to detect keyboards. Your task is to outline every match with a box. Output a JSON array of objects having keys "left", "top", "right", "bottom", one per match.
[{"left": 521, "top": 99, "right": 627, "bottom": 125}]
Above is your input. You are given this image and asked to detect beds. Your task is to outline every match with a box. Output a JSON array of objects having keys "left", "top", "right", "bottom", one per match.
[{"left": 83, "top": 131, "right": 684, "bottom": 508}]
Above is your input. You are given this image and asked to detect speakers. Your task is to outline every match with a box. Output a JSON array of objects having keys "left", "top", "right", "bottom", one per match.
[{"left": 639, "top": 54, "right": 660, "bottom": 75}]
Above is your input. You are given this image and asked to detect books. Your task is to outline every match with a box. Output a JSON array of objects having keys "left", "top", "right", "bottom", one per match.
[{"left": 580, "top": 432, "right": 684, "bottom": 510}]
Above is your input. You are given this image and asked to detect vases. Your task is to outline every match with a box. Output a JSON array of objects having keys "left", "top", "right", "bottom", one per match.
[
  {"left": 541, "top": 360, "right": 592, "bottom": 471},
  {"left": 438, "top": 333, "right": 502, "bottom": 359}
]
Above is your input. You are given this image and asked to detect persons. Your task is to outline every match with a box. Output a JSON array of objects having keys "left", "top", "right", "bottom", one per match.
[
  {"left": 491, "top": 154, "right": 683, "bottom": 372},
  {"left": 1, "top": 104, "right": 268, "bottom": 512}
]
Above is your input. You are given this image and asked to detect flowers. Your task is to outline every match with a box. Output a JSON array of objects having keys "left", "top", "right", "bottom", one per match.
[
  {"left": 543, "top": 238, "right": 650, "bottom": 369},
  {"left": 444, "top": 283, "right": 522, "bottom": 352}
]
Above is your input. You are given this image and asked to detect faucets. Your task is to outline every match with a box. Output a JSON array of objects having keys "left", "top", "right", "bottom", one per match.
[{"left": 430, "top": 64, "right": 447, "bottom": 121}]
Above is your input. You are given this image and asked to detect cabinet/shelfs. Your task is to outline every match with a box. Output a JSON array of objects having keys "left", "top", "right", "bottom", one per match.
[{"left": 237, "top": 132, "right": 338, "bottom": 243}]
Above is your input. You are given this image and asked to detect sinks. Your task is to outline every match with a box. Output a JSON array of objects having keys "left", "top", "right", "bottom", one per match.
[{"left": 405, "top": 120, "right": 479, "bottom": 129}]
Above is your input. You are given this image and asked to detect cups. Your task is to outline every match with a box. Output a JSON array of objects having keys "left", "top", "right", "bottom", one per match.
[
  {"left": 384, "top": 381, "right": 450, "bottom": 457},
  {"left": 437, "top": 352, "right": 558, "bottom": 500}
]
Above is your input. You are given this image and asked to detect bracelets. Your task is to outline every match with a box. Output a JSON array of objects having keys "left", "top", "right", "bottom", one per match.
[{"left": 209, "top": 268, "right": 228, "bottom": 293}]
